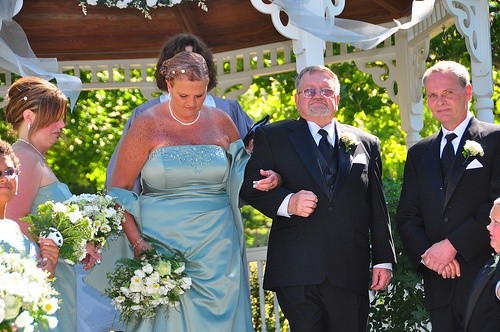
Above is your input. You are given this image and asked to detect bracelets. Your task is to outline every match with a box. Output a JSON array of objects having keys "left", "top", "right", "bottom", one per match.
[{"left": 132, "top": 238, "right": 143, "bottom": 249}]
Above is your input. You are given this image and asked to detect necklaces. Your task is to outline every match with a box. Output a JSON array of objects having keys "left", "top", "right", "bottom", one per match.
[
  {"left": 169, "top": 99, "right": 201, "bottom": 125},
  {"left": 17, "top": 138, "right": 47, "bottom": 160}
]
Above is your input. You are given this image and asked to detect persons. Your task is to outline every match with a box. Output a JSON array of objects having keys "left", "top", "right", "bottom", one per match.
[
  {"left": 6, "top": 77, "right": 101, "bottom": 332},
  {"left": 238, "top": 65, "right": 396, "bottom": 332},
  {"left": 396, "top": 62, "right": 500, "bottom": 332},
  {"left": 462, "top": 199, "right": 500, "bottom": 332},
  {"left": 0, "top": 138, "right": 60, "bottom": 332},
  {"left": 105, "top": 34, "right": 255, "bottom": 195},
  {"left": 108, "top": 53, "right": 279, "bottom": 332}
]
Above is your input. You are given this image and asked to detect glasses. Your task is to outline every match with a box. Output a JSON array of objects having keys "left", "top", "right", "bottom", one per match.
[{"left": 296, "top": 88, "right": 338, "bottom": 99}]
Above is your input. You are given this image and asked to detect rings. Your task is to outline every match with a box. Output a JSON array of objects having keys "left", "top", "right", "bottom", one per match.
[
  {"left": 266, "top": 171, "right": 269, "bottom": 176},
  {"left": 266, "top": 190, "right": 268, "bottom": 192},
  {"left": 271, "top": 183, "right": 274, "bottom": 188}
]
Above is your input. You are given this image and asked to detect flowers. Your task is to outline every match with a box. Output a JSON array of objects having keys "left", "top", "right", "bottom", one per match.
[
  {"left": 0, "top": 188, "right": 192, "bottom": 332},
  {"left": 78, "top": 0, "right": 208, "bottom": 20},
  {"left": 462, "top": 140, "right": 484, "bottom": 165},
  {"left": 340, "top": 131, "right": 357, "bottom": 153}
]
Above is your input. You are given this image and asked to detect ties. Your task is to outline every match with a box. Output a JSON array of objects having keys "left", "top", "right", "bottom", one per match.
[
  {"left": 317, "top": 129, "right": 335, "bottom": 164},
  {"left": 442, "top": 133, "right": 458, "bottom": 180}
]
